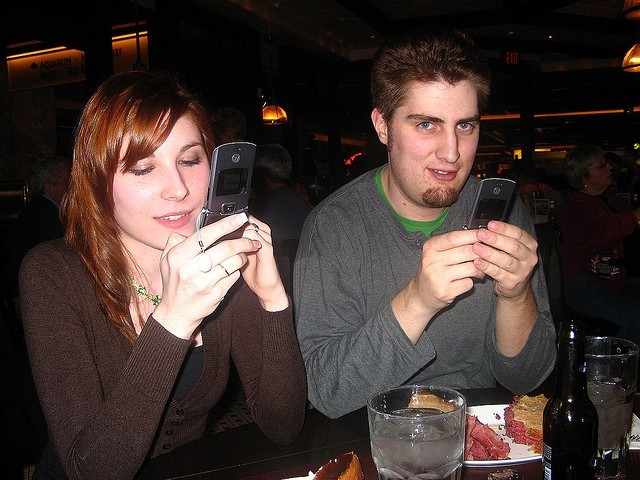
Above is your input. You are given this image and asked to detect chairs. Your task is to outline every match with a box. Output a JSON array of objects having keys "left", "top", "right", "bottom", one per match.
[{"left": 544, "top": 210, "right": 621, "bottom": 404}]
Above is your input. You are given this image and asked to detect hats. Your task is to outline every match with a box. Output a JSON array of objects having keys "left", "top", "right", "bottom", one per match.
[{"left": 256, "top": 142, "right": 293, "bottom": 182}]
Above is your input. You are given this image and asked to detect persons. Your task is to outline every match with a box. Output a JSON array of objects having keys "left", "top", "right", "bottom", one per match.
[
  {"left": 245, "top": 142, "right": 314, "bottom": 296},
  {"left": 628, "top": 162, "right": 639, "bottom": 196},
  {"left": 559, "top": 142, "right": 639, "bottom": 340},
  {"left": 17, "top": 69, "right": 308, "bottom": 477},
  {"left": 604, "top": 151, "right": 622, "bottom": 181},
  {"left": 9, "top": 154, "right": 73, "bottom": 324},
  {"left": 292, "top": 27, "right": 559, "bottom": 419},
  {"left": 293, "top": 177, "right": 315, "bottom": 207},
  {"left": 508, "top": 155, "right": 562, "bottom": 217},
  {"left": 210, "top": 106, "right": 246, "bottom": 144}
]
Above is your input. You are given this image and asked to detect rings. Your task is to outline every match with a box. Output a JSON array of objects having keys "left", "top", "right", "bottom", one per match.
[
  {"left": 219, "top": 262, "right": 233, "bottom": 275},
  {"left": 252, "top": 223, "right": 260, "bottom": 232}
]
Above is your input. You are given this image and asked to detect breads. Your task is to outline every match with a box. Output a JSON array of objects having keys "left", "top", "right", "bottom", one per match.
[
  {"left": 313, "top": 452, "right": 362, "bottom": 480},
  {"left": 510, "top": 393, "right": 548, "bottom": 432}
]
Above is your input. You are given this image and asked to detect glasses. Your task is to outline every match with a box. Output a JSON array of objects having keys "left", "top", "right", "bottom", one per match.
[{"left": 591, "top": 159, "right": 610, "bottom": 169}]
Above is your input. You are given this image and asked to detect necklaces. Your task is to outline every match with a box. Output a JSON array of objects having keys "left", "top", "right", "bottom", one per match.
[{"left": 122, "top": 270, "right": 162, "bottom": 308}]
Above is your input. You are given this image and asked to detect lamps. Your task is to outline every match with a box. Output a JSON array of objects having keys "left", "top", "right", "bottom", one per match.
[
  {"left": 621, "top": 42, "right": 640, "bottom": 73},
  {"left": 262, "top": 35, "right": 288, "bottom": 125}
]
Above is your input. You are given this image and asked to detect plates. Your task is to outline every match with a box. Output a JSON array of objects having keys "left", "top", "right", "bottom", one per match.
[
  {"left": 629, "top": 411, "right": 640, "bottom": 452},
  {"left": 463, "top": 403, "right": 544, "bottom": 467}
]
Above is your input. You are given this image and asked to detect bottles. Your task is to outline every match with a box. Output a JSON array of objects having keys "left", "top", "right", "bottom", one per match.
[{"left": 541, "top": 320, "right": 599, "bottom": 480}]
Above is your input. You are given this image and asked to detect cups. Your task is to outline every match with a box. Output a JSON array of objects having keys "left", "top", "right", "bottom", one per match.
[
  {"left": 581, "top": 335, "right": 640, "bottom": 480},
  {"left": 367, "top": 385, "right": 467, "bottom": 480}
]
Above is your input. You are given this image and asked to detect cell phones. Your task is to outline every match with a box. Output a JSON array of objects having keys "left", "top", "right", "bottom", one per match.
[
  {"left": 201, "top": 141, "right": 256, "bottom": 254},
  {"left": 466, "top": 178, "right": 516, "bottom": 249}
]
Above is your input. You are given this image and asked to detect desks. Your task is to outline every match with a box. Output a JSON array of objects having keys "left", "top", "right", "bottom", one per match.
[{"left": 132, "top": 386, "right": 639, "bottom": 480}]
